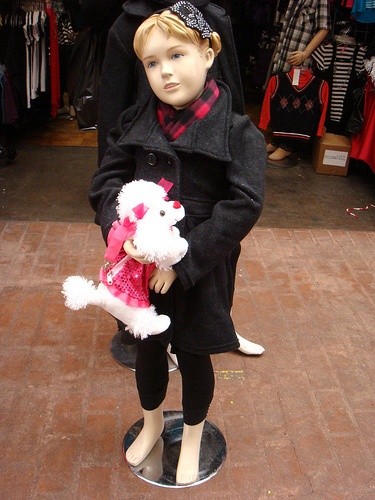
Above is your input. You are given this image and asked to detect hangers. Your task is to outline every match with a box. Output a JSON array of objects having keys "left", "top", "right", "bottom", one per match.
[
  {"left": 0, "top": 0, "right": 45, "bottom": 27},
  {"left": 334, "top": 21, "right": 356, "bottom": 46},
  {"left": 296, "top": 52, "right": 307, "bottom": 71}
]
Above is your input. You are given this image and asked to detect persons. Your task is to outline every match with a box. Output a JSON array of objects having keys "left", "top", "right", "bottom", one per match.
[
  {"left": 263, "top": 0, "right": 332, "bottom": 161},
  {"left": 88, "top": 0, "right": 267, "bottom": 485},
  {"left": 97, "top": 0, "right": 268, "bottom": 369}
]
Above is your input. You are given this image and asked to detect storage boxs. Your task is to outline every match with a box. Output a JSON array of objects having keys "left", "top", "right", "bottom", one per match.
[{"left": 314, "top": 133, "right": 351, "bottom": 176}]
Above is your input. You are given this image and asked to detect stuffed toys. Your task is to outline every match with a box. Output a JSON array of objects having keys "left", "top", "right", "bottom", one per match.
[{"left": 62, "top": 178, "right": 188, "bottom": 338}]
